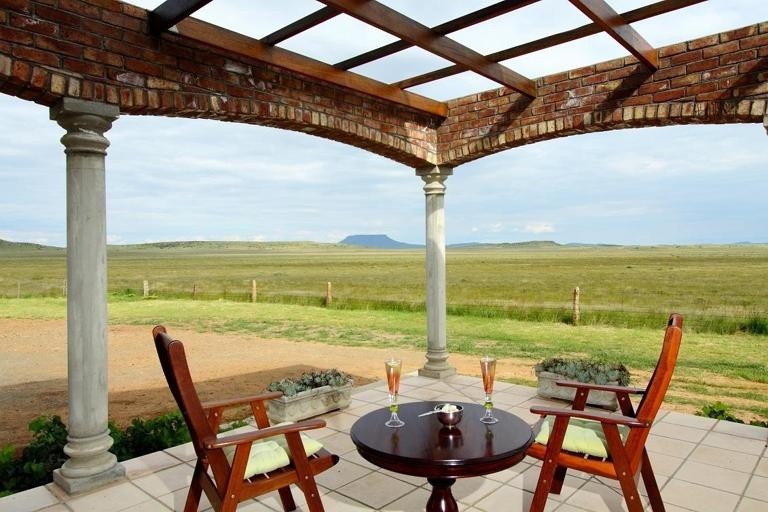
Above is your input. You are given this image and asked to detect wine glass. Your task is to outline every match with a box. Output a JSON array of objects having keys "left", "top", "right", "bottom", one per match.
[
  {"left": 381, "top": 355, "right": 405, "bottom": 429},
  {"left": 478, "top": 356, "right": 499, "bottom": 425}
]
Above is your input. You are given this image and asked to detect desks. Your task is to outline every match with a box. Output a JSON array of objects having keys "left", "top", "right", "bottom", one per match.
[{"left": 348, "top": 397, "right": 539, "bottom": 511}]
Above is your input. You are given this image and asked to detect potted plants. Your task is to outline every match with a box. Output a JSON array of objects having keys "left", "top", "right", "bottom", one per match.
[
  {"left": 531, "top": 353, "right": 631, "bottom": 412},
  {"left": 259, "top": 366, "right": 351, "bottom": 423}
]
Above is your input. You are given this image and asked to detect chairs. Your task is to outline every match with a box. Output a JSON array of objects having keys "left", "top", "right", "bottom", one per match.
[
  {"left": 525, "top": 313, "right": 686, "bottom": 511},
  {"left": 141, "top": 323, "right": 343, "bottom": 512}
]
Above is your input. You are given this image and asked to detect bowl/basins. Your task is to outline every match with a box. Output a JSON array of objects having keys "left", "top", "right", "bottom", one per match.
[{"left": 432, "top": 402, "right": 463, "bottom": 428}]
